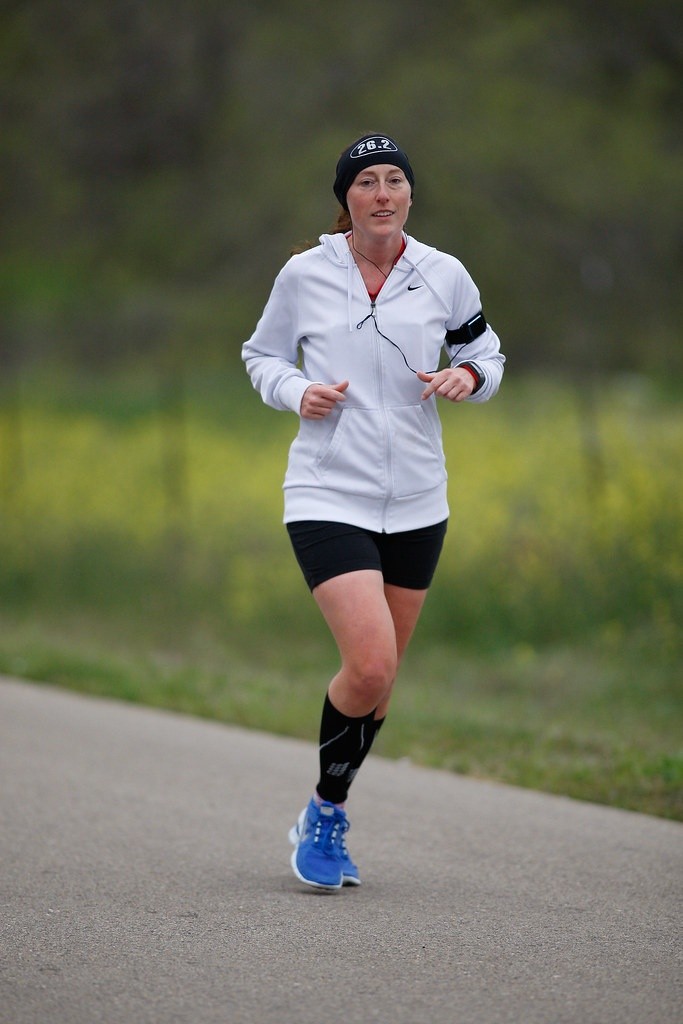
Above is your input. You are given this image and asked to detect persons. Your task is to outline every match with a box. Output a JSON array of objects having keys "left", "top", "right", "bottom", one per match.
[{"left": 240, "top": 132, "right": 507, "bottom": 891}]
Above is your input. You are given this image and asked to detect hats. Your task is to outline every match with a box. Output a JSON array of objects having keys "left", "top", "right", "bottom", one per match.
[{"left": 334, "top": 135, "right": 415, "bottom": 210}]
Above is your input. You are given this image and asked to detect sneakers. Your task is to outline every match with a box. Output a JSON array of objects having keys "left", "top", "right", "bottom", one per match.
[{"left": 288, "top": 796, "right": 361, "bottom": 888}]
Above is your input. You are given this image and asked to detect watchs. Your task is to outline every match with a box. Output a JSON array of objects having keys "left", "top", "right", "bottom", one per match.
[{"left": 456, "top": 361, "right": 486, "bottom": 395}]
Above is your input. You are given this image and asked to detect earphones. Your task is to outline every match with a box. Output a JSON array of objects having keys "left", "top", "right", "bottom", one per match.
[{"left": 410, "top": 191, "right": 414, "bottom": 199}]
